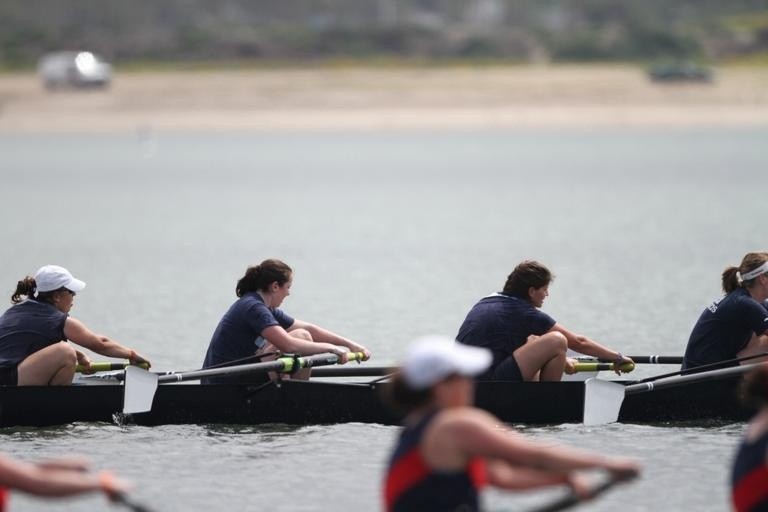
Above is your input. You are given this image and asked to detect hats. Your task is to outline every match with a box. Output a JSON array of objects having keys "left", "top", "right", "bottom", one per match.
[
  {"left": 32, "top": 264, "right": 86, "bottom": 293},
  {"left": 402, "top": 334, "right": 494, "bottom": 390}
]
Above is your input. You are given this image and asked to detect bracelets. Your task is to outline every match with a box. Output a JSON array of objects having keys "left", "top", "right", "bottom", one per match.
[
  {"left": 613, "top": 351, "right": 622, "bottom": 364},
  {"left": 130, "top": 350, "right": 136, "bottom": 361},
  {"left": 101, "top": 474, "right": 113, "bottom": 496}
]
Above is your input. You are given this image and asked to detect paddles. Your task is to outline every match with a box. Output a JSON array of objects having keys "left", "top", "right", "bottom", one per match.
[
  {"left": 122, "top": 353, "right": 363, "bottom": 416},
  {"left": 584, "top": 362, "right": 763, "bottom": 425}
]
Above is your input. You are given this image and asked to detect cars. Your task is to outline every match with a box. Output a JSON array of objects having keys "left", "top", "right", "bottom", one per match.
[
  {"left": 644, "top": 48, "right": 724, "bottom": 87},
  {"left": 32, "top": 45, "right": 116, "bottom": 94}
]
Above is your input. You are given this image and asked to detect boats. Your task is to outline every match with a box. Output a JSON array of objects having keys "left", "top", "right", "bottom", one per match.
[{"left": 0, "top": 341, "right": 767, "bottom": 430}]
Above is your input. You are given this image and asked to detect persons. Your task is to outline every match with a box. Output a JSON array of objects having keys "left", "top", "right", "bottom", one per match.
[
  {"left": 680, "top": 252, "right": 768, "bottom": 376},
  {"left": 0, "top": 452, "right": 137, "bottom": 512},
  {"left": 0, "top": 264, "right": 151, "bottom": 386},
  {"left": 201, "top": 259, "right": 371, "bottom": 386},
  {"left": 731, "top": 364, "right": 768, "bottom": 512},
  {"left": 383, "top": 337, "right": 641, "bottom": 512},
  {"left": 456, "top": 260, "right": 635, "bottom": 382}
]
